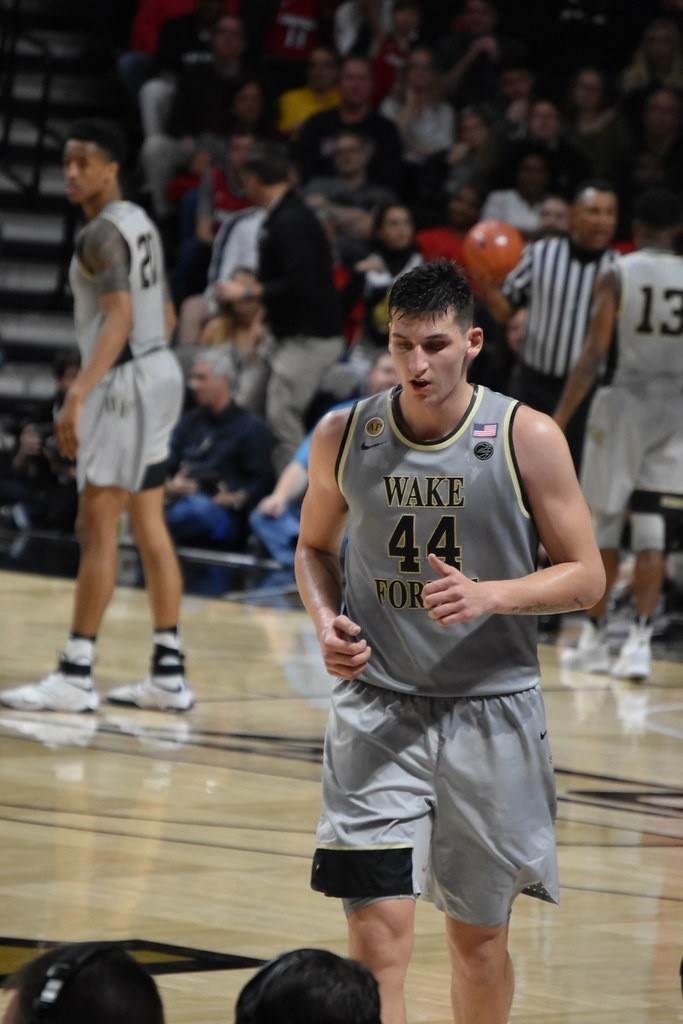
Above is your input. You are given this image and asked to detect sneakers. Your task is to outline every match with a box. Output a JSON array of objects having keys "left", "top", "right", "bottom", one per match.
[
  {"left": 605, "top": 638, "right": 653, "bottom": 681},
  {"left": 1, "top": 670, "right": 102, "bottom": 713},
  {"left": 555, "top": 636, "right": 614, "bottom": 672},
  {"left": 104, "top": 674, "right": 196, "bottom": 712}
]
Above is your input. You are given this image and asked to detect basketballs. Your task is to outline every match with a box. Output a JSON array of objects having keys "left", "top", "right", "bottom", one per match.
[{"left": 464, "top": 219, "right": 520, "bottom": 274}]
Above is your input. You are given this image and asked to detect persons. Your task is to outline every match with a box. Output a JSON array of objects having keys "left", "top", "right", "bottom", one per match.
[
  {"left": 234, "top": 948, "right": 383, "bottom": 1024},
  {"left": 0, "top": 942, "right": 165, "bottom": 1024},
  {"left": 0, "top": 0, "right": 683, "bottom": 714},
  {"left": 293, "top": 260, "right": 606, "bottom": 1024}
]
